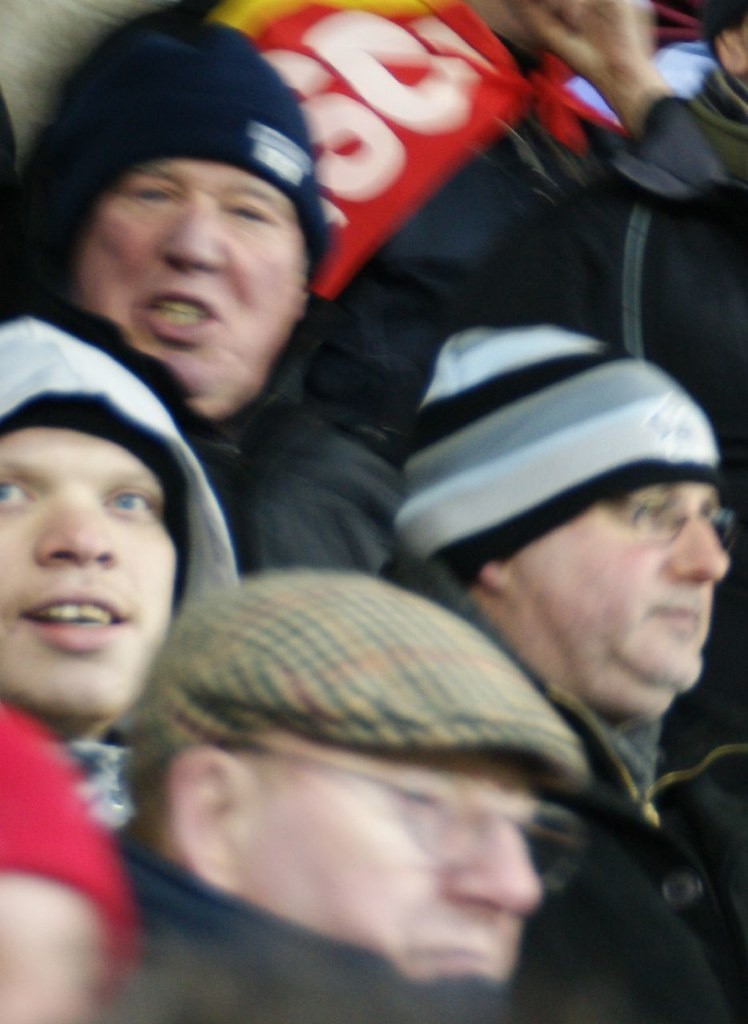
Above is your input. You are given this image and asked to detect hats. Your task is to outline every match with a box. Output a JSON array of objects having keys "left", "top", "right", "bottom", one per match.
[
  {"left": 391, "top": 321, "right": 722, "bottom": 586},
  {"left": 121, "top": 568, "right": 590, "bottom": 787},
  {"left": 0, "top": 391, "right": 187, "bottom": 611},
  {"left": 22, "top": 10, "right": 328, "bottom": 312}
]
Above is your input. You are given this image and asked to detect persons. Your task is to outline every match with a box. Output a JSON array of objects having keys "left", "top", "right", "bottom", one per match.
[{"left": 0, "top": 1, "right": 748, "bottom": 1024}]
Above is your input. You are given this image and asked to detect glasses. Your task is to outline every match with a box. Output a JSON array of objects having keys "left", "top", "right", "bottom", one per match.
[
  {"left": 573, "top": 480, "right": 739, "bottom": 556},
  {"left": 217, "top": 730, "right": 593, "bottom": 888}
]
